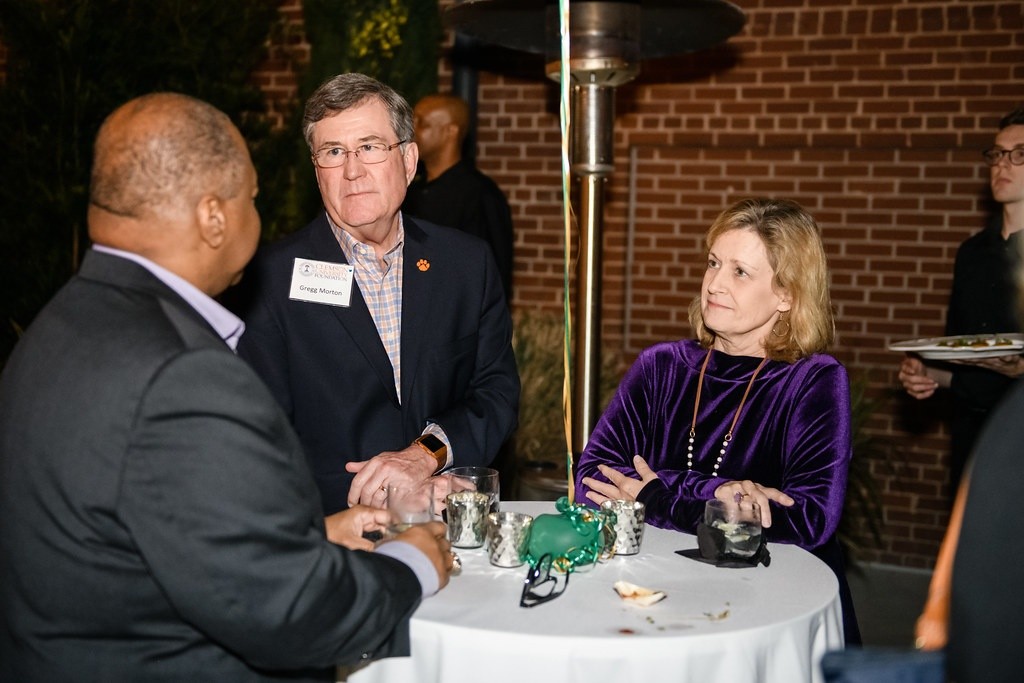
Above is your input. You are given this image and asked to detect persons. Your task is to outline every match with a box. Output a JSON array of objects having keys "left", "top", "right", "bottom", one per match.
[
  {"left": 407, "top": 93, "right": 521, "bottom": 502},
  {"left": 226, "top": 70, "right": 523, "bottom": 532},
  {"left": 940, "top": 227, "right": 1024, "bottom": 682},
  {"left": 574, "top": 195, "right": 864, "bottom": 651},
  {"left": 889, "top": 108, "right": 1024, "bottom": 553},
  {"left": 0, "top": 92, "right": 451, "bottom": 682}
]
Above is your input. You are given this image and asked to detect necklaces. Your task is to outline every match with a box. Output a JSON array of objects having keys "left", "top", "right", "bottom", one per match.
[{"left": 686, "top": 332, "right": 775, "bottom": 477}]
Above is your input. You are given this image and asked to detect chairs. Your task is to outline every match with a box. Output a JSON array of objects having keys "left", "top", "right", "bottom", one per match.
[{"left": 822, "top": 392, "right": 1023, "bottom": 683}]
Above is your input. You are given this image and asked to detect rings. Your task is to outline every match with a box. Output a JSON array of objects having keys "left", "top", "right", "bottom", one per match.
[
  {"left": 378, "top": 485, "right": 388, "bottom": 492},
  {"left": 733, "top": 492, "right": 749, "bottom": 502}
]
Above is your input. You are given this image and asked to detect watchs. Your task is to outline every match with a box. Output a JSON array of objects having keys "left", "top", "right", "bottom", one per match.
[{"left": 410, "top": 432, "right": 447, "bottom": 474}]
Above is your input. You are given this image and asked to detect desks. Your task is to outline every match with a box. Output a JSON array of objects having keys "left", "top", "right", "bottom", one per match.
[{"left": 335, "top": 501, "right": 845, "bottom": 683}]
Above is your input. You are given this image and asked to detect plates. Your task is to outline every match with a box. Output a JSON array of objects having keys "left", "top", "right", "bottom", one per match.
[{"left": 889, "top": 332, "right": 1024, "bottom": 361}]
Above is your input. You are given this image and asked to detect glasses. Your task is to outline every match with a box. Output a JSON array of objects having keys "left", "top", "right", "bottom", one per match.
[
  {"left": 982, "top": 148, "right": 1024, "bottom": 166},
  {"left": 311, "top": 139, "right": 407, "bottom": 168},
  {"left": 519, "top": 552, "right": 570, "bottom": 607}
]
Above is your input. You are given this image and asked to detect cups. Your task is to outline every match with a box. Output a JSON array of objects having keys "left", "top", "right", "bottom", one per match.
[
  {"left": 485, "top": 512, "right": 533, "bottom": 568},
  {"left": 443, "top": 467, "right": 500, "bottom": 522},
  {"left": 704, "top": 499, "right": 762, "bottom": 560},
  {"left": 387, "top": 479, "right": 435, "bottom": 537},
  {"left": 600, "top": 499, "right": 645, "bottom": 556},
  {"left": 445, "top": 492, "right": 490, "bottom": 549}
]
